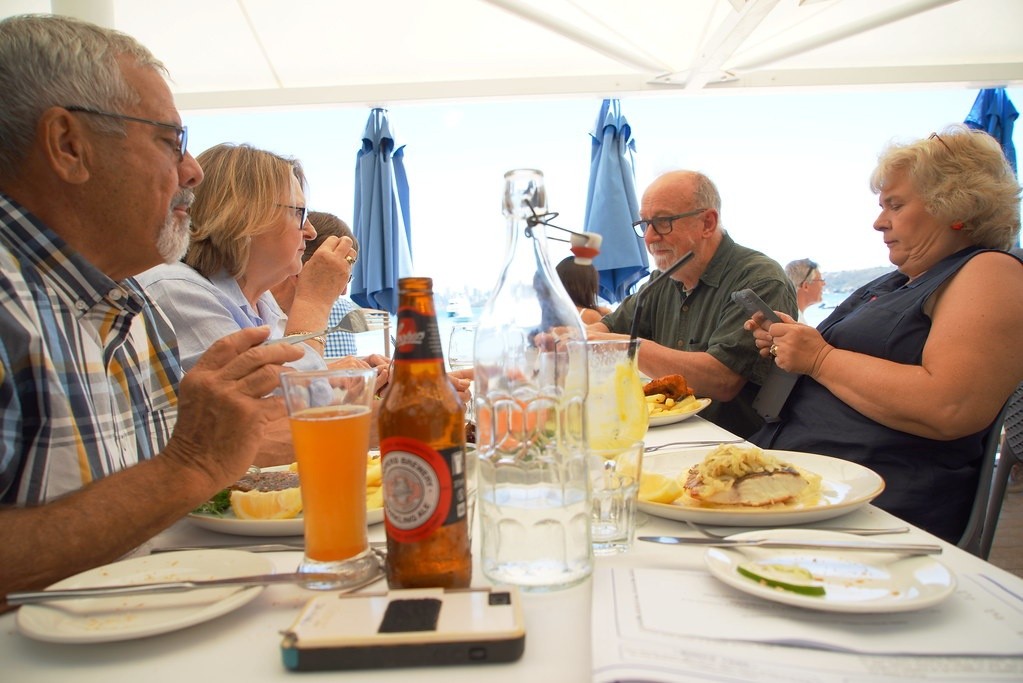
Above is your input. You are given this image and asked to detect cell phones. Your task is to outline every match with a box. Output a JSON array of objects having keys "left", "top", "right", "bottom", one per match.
[
  {"left": 278, "top": 586, "right": 525, "bottom": 672},
  {"left": 730, "top": 288, "right": 784, "bottom": 331}
]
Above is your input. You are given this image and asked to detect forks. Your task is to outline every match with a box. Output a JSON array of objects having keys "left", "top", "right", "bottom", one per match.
[
  {"left": 249, "top": 309, "right": 392, "bottom": 345},
  {"left": 686, "top": 521, "right": 910, "bottom": 540},
  {"left": 389, "top": 335, "right": 397, "bottom": 373},
  {"left": 645, "top": 437, "right": 748, "bottom": 452}
]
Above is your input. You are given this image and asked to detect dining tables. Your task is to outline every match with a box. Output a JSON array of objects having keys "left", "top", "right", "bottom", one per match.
[{"left": 0, "top": 375, "right": 1022, "bottom": 681}]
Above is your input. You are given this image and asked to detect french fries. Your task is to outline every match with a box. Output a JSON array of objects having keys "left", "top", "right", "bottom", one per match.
[
  {"left": 288, "top": 454, "right": 386, "bottom": 511},
  {"left": 646, "top": 393, "right": 701, "bottom": 418}
]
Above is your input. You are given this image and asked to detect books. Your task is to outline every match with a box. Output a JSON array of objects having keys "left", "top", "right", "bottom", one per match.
[
  {"left": 348, "top": 109, "right": 414, "bottom": 356},
  {"left": 963, "top": 88, "right": 1023, "bottom": 248},
  {"left": 587, "top": 97, "right": 651, "bottom": 307}
]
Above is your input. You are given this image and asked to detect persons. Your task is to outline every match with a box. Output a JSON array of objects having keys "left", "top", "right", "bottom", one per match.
[
  {"left": 0, "top": 14, "right": 390, "bottom": 628},
  {"left": 744, "top": 117, "right": 1023, "bottom": 546},
  {"left": 785, "top": 259, "right": 826, "bottom": 325},
  {"left": 134, "top": 142, "right": 504, "bottom": 418},
  {"left": 527, "top": 170, "right": 798, "bottom": 442}
]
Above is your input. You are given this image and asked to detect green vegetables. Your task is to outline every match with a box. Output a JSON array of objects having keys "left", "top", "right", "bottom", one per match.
[{"left": 193, "top": 490, "right": 231, "bottom": 518}]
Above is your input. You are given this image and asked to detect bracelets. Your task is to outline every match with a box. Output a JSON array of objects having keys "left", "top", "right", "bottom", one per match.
[{"left": 288, "top": 330, "right": 326, "bottom": 344}]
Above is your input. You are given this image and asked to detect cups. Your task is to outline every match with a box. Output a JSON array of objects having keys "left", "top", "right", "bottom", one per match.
[
  {"left": 449, "top": 325, "right": 476, "bottom": 371},
  {"left": 540, "top": 351, "right": 568, "bottom": 397},
  {"left": 279, "top": 368, "right": 379, "bottom": 591},
  {"left": 560, "top": 339, "right": 649, "bottom": 555}
]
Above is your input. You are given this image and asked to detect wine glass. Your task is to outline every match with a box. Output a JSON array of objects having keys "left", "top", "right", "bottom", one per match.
[{"left": 503, "top": 331, "right": 523, "bottom": 394}]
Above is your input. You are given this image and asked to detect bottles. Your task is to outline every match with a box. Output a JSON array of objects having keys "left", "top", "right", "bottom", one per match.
[
  {"left": 379, "top": 278, "right": 472, "bottom": 591},
  {"left": 475, "top": 168, "right": 596, "bottom": 593}
]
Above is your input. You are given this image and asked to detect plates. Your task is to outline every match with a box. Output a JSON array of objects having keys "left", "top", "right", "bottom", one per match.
[
  {"left": 648, "top": 399, "right": 711, "bottom": 427},
  {"left": 636, "top": 449, "right": 886, "bottom": 526},
  {"left": 185, "top": 451, "right": 385, "bottom": 536},
  {"left": 705, "top": 528, "right": 957, "bottom": 614},
  {"left": 16, "top": 549, "right": 273, "bottom": 645}
]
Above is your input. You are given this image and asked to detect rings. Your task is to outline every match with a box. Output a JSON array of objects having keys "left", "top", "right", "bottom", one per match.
[
  {"left": 770, "top": 344, "right": 778, "bottom": 356},
  {"left": 346, "top": 256, "right": 356, "bottom": 264}
]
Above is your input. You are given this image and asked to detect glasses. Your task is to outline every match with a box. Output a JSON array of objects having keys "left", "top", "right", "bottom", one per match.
[
  {"left": 632, "top": 211, "right": 702, "bottom": 238},
  {"left": 67, "top": 105, "right": 188, "bottom": 161},
  {"left": 279, "top": 205, "right": 307, "bottom": 229},
  {"left": 930, "top": 132, "right": 957, "bottom": 157},
  {"left": 801, "top": 262, "right": 817, "bottom": 286}
]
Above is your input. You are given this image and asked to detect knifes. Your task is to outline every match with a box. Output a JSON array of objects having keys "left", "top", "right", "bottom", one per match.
[
  {"left": 151, "top": 543, "right": 386, "bottom": 554},
  {"left": 7, "top": 573, "right": 353, "bottom": 607},
  {"left": 638, "top": 537, "right": 942, "bottom": 554}
]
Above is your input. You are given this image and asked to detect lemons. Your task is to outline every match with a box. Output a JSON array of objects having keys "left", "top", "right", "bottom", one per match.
[
  {"left": 637, "top": 472, "right": 684, "bottom": 504},
  {"left": 228, "top": 487, "right": 303, "bottom": 519}
]
